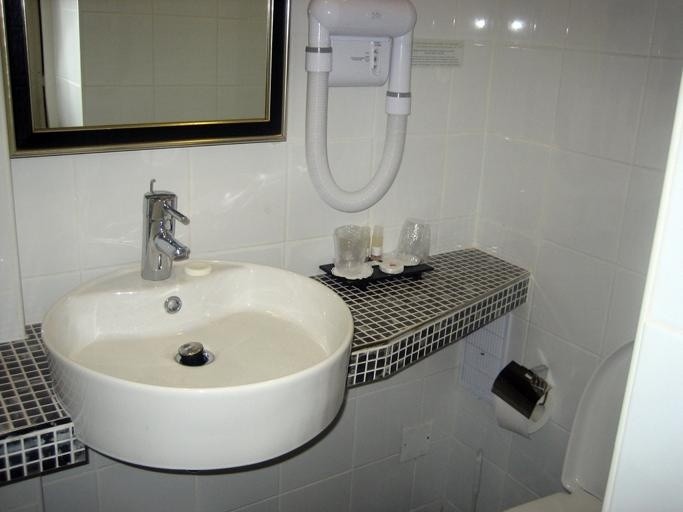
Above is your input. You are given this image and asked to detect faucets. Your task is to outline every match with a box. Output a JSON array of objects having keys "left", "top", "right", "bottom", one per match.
[{"left": 141, "top": 192, "right": 192, "bottom": 282}]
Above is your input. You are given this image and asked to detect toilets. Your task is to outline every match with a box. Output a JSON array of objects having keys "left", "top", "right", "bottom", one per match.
[{"left": 499, "top": 340, "right": 637, "bottom": 511}]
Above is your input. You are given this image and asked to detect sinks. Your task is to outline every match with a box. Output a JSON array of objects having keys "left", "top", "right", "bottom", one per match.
[{"left": 40, "top": 257, "right": 354, "bottom": 469}]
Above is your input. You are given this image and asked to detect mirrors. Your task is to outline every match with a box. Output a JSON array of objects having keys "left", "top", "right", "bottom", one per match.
[{"left": 0, "top": 0, "right": 288, "bottom": 159}]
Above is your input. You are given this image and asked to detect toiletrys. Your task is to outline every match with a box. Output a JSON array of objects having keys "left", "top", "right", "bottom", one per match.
[{"left": 362, "top": 226, "right": 370, "bottom": 262}]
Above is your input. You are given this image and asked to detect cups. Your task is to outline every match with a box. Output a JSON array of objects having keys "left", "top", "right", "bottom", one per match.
[
  {"left": 336, "top": 225, "right": 369, "bottom": 272},
  {"left": 396, "top": 220, "right": 431, "bottom": 262}
]
